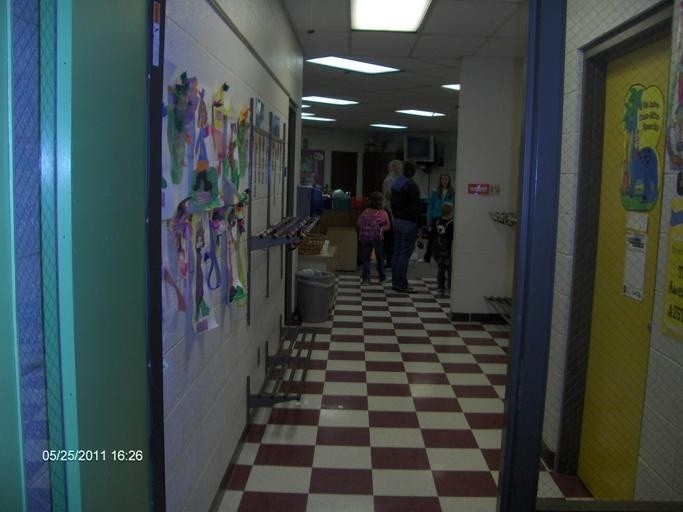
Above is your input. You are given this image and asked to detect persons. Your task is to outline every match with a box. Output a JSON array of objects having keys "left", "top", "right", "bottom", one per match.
[
  {"left": 423, "top": 173, "right": 454, "bottom": 230},
  {"left": 390, "top": 161, "right": 424, "bottom": 294},
  {"left": 423, "top": 202, "right": 454, "bottom": 298},
  {"left": 355, "top": 189, "right": 388, "bottom": 286},
  {"left": 380, "top": 158, "right": 402, "bottom": 272}
]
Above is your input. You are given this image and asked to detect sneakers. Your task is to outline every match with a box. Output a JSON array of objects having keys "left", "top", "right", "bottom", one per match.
[
  {"left": 437, "top": 289, "right": 451, "bottom": 296},
  {"left": 392, "top": 286, "right": 419, "bottom": 294},
  {"left": 359, "top": 277, "right": 388, "bottom": 285},
  {"left": 418, "top": 258, "right": 426, "bottom": 263}
]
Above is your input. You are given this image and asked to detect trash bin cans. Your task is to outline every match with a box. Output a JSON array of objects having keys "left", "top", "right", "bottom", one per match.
[{"left": 296, "top": 269, "right": 335, "bottom": 322}]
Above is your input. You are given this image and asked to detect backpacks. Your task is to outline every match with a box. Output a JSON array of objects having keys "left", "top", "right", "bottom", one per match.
[{"left": 358, "top": 208, "right": 385, "bottom": 245}]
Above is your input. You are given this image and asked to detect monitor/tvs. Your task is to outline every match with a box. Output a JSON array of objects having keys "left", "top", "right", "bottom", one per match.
[{"left": 404, "top": 135, "right": 433, "bottom": 162}]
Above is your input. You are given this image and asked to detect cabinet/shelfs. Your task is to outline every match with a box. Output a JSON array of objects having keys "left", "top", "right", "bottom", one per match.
[
  {"left": 297, "top": 245, "right": 339, "bottom": 274},
  {"left": 245, "top": 215, "right": 322, "bottom": 405}
]
